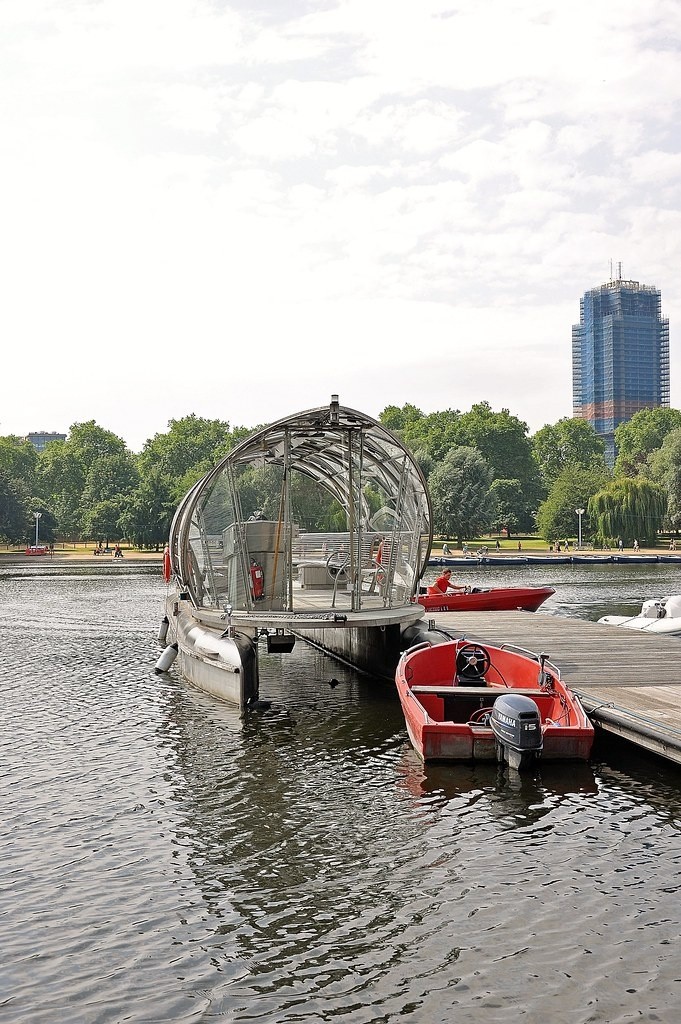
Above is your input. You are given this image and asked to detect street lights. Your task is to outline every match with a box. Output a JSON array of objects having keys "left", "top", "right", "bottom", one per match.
[
  {"left": 575, "top": 508, "right": 585, "bottom": 546},
  {"left": 32, "top": 512, "right": 42, "bottom": 549}
]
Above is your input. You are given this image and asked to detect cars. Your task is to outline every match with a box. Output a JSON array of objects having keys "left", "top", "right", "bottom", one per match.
[{"left": 29, "top": 544, "right": 45, "bottom": 549}]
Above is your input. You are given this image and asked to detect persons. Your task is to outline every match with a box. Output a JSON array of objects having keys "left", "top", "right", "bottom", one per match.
[
  {"left": 443, "top": 543, "right": 448, "bottom": 555},
  {"left": 633, "top": 539, "right": 640, "bottom": 552},
  {"left": 441, "top": 535, "right": 444, "bottom": 541},
  {"left": 463, "top": 543, "right": 489, "bottom": 556},
  {"left": 669, "top": 539, "right": 677, "bottom": 550},
  {"left": 554, "top": 540, "right": 579, "bottom": 554},
  {"left": 95, "top": 546, "right": 106, "bottom": 556},
  {"left": 496, "top": 540, "right": 500, "bottom": 548},
  {"left": 113, "top": 545, "right": 123, "bottom": 558},
  {"left": 618, "top": 539, "right": 623, "bottom": 551},
  {"left": 428, "top": 568, "right": 466, "bottom": 595},
  {"left": 518, "top": 542, "right": 521, "bottom": 549}
]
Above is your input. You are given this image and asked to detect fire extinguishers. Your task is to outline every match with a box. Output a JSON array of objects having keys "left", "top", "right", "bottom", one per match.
[{"left": 248, "top": 557, "right": 265, "bottom": 602}]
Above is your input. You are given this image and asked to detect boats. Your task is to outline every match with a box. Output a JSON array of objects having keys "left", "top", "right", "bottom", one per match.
[
  {"left": 597, "top": 595, "right": 681, "bottom": 637},
  {"left": 611, "top": 556, "right": 657, "bottom": 563},
  {"left": 526, "top": 556, "right": 571, "bottom": 564},
  {"left": 396, "top": 633, "right": 595, "bottom": 771},
  {"left": 658, "top": 555, "right": 681, "bottom": 563},
  {"left": 571, "top": 555, "right": 612, "bottom": 564},
  {"left": 412, "top": 585, "right": 556, "bottom": 612},
  {"left": 477, "top": 557, "right": 526, "bottom": 565},
  {"left": 440, "top": 557, "right": 479, "bottom": 566},
  {"left": 413, "top": 557, "right": 439, "bottom": 566}
]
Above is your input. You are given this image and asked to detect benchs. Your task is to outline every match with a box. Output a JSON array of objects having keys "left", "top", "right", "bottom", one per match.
[
  {"left": 410, "top": 685, "right": 550, "bottom": 697},
  {"left": 332, "top": 533, "right": 388, "bottom": 596},
  {"left": 94, "top": 548, "right": 112, "bottom": 556}
]
